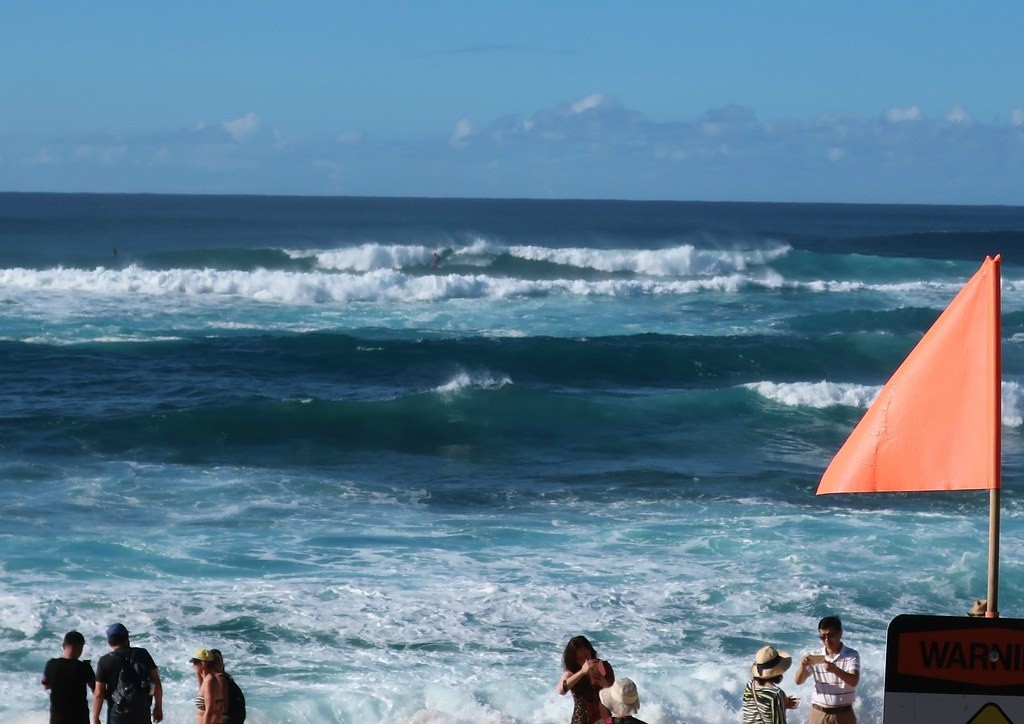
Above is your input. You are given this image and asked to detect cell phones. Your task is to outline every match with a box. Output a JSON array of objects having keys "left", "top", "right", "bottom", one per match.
[
  {"left": 82, "top": 658, "right": 91, "bottom": 667},
  {"left": 808, "top": 655, "right": 825, "bottom": 664},
  {"left": 589, "top": 664, "right": 600, "bottom": 685}
]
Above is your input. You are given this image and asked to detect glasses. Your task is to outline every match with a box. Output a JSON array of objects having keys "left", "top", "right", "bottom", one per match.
[{"left": 819, "top": 632, "right": 839, "bottom": 640}]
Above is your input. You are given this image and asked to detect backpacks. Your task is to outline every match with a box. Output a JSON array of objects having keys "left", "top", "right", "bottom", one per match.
[
  {"left": 106, "top": 647, "right": 155, "bottom": 715},
  {"left": 219, "top": 672, "right": 246, "bottom": 724}
]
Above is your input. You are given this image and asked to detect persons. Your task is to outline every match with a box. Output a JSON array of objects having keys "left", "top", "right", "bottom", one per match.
[
  {"left": 554, "top": 636, "right": 619, "bottom": 724},
  {"left": 40, "top": 630, "right": 104, "bottom": 724},
  {"left": 91, "top": 622, "right": 165, "bottom": 724},
  {"left": 592, "top": 677, "right": 655, "bottom": 724},
  {"left": 187, "top": 647, "right": 223, "bottom": 724},
  {"left": 738, "top": 645, "right": 803, "bottom": 724},
  {"left": 795, "top": 613, "right": 862, "bottom": 724},
  {"left": 202, "top": 650, "right": 245, "bottom": 724}
]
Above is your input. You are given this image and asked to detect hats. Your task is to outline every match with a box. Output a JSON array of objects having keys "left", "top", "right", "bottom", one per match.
[
  {"left": 106, "top": 623, "right": 130, "bottom": 641},
  {"left": 964, "top": 600, "right": 989, "bottom": 617},
  {"left": 599, "top": 677, "right": 641, "bottom": 716},
  {"left": 188, "top": 650, "right": 213, "bottom": 664},
  {"left": 751, "top": 646, "right": 792, "bottom": 679}
]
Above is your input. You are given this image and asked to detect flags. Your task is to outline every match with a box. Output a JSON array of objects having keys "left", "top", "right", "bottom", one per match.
[{"left": 813, "top": 251, "right": 1006, "bottom": 496}]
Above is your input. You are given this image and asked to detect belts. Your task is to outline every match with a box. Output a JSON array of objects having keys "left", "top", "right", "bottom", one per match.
[{"left": 812, "top": 704, "right": 853, "bottom": 714}]
